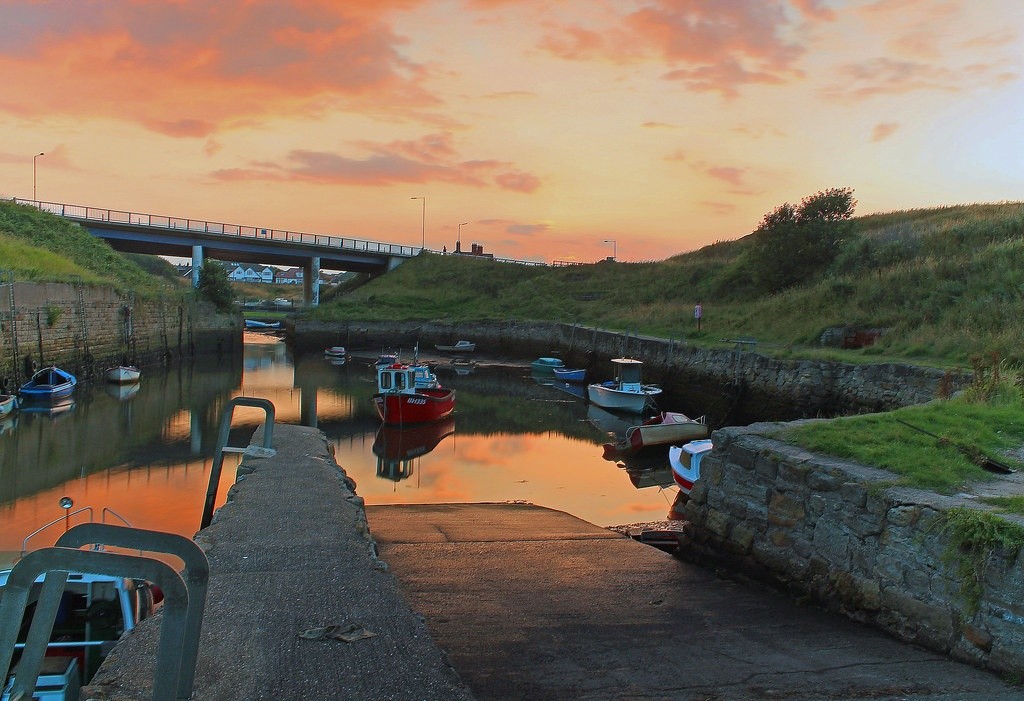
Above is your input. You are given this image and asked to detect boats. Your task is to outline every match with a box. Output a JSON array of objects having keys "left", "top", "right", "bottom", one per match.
[
  {"left": 373, "top": 370, "right": 456, "bottom": 426},
  {"left": 587, "top": 357, "right": 663, "bottom": 416},
  {"left": 324, "top": 346, "right": 346, "bottom": 356},
  {"left": 531, "top": 357, "right": 564, "bottom": 372},
  {"left": 603, "top": 410, "right": 708, "bottom": 462},
  {"left": 105, "top": 384, "right": 140, "bottom": 401},
  {"left": 0, "top": 393, "right": 16, "bottom": 421},
  {"left": 105, "top": 365, "right": 141, "bottom": 383},
  {"left": 668, "top": 438, "right": 713, "bottom": 493},
  {"left": 272, "top": 297, "right": 293, "bottom": 305},
  {"left": 245, "top": 318, "right": 281, "bottom": 329},
  {"left": 17, "top": 365, "right": 77, "bottom": 403},
  {"left": 434, "top": 342, "right": 477, "bottom": 352},
  {"left": 552, "top": 367, "right": 587, "bottom": 381},
  {"left": 373, "top": 355, "right": 400, "bottom": 369},
  {"left": 1, "top": 550, "right": 170, "bottom": 701}
]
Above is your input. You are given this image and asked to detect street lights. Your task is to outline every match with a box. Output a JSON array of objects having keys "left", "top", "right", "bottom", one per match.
[
  {"left": 458, "top": 223, "right": 467, "bottom": 254},
  {"left": 32, "top": 152, "right": 43, "bottom": 212},
  {"left": 604, "top": 240, "right": 616, "bottom": 261},
  {"left": 410, "top": 197, "right": 425, "bottom": 248}
]
